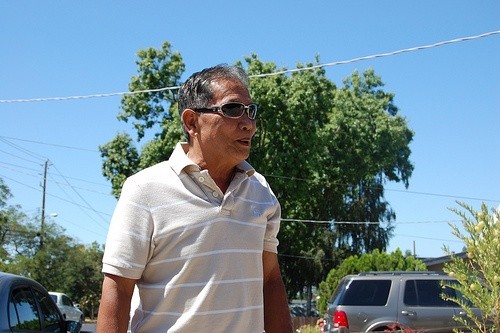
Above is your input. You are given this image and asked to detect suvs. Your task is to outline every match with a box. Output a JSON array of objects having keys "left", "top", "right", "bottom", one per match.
[{"left": 325, "top": 268, "right": 496, "bottom": 333}]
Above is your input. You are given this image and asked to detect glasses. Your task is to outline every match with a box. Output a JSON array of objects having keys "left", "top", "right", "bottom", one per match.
[{"left": 193, "top": 101, "right": 257, "bottom": 120}]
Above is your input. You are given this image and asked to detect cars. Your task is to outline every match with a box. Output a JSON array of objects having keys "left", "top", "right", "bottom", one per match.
[
  {"left": 0, "top": 271, "right": 83, "bottom": 332},
  {"left": 48, "top": 291, "right": 84, "bottom": 324}
]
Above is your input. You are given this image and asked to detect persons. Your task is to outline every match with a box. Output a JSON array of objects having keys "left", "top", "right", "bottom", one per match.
[
  {"left": 317, "top": 319, "right": 325, "bottom": 332},
  {"left": 96, "top": 64, "right": 293, "bottom": 333}
]
